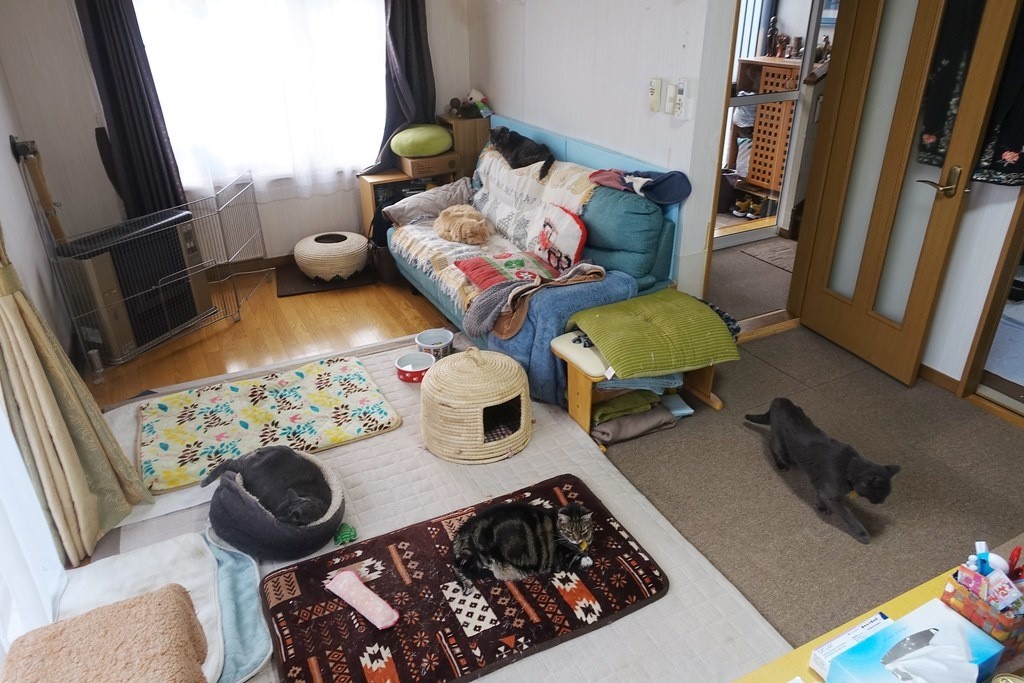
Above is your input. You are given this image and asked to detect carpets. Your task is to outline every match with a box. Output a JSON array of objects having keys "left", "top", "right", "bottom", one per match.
[
  {"left": 88, "top": 328, "right": 795, "bottom": 683},
  {"left": 739, "top": 238, "right": 797, "bottom": 273},
  {"left": 276, "top": 263, "right": 376, "bottom": 297}
]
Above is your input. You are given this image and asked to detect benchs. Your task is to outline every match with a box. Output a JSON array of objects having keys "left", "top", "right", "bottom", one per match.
[{"left": 550, "top": 330, "right": 724, "bottom": 454}]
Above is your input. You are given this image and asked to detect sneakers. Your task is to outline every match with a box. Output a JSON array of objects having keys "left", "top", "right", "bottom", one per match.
[
  {"left": 732, "top": 196, "right": 753, "bottom": 218},
  {"left": 747, "top": 196, "right": 764, "bottom": 219}
]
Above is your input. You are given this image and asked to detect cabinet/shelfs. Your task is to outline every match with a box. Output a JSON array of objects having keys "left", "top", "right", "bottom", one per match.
[
  {"left": 437, "top": 113, "right": 490, "bottom": 182},
  {"left": 726, "top": 54, "right": 820, "bottom": 202},
  {"left": 359, "top": 167, "right": 453, "bottom": 238}
]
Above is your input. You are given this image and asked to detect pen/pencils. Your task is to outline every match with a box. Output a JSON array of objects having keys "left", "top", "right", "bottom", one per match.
[{"left": 1009, "top": 545, "right": 1021, "bottom": 577}]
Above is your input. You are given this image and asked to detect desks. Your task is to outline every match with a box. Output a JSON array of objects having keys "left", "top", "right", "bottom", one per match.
[{"left": 737, "top": 566, "right": 1024, "bottom": 683}]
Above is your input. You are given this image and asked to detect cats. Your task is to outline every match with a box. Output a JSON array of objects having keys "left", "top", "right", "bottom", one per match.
[
  {"left": 432, "top": 204, "right": 496, "bottom": 245},
  {"left": 452, "top": 502, "right": 595, "bottom": 582},
  {"left": 198, "top": 445, "right": 333, "bottom": 527},
  {"left": 488, "top": 126, "right": 556, "bottom": 181},
  {"left": 743, "top": 397, "right": 902, "bottom": 546}
]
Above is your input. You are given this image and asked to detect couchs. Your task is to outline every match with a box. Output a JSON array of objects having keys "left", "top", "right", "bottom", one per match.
[{"left": 382, "top": 141, "right": 675, "bottom": 407}]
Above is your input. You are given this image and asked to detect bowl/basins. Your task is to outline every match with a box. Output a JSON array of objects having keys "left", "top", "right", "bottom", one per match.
[
  {"left": 395, "top": 352, "right": 436, "bottom": 383},
  {"left": 415, "top": 328, "right": 454, "bottom": 361}
]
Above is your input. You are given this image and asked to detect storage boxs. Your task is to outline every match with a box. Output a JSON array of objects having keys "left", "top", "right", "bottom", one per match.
[
  {"left": 826, "top": 597, "right": 1005, "bottom": 683},
  {"left": 368, "top": 236, "right": 403, "bottom": 282},
  {"left": 943, "top": 570, "right": 1024, "bottom": 663},
  {"left": 391, "top": 151, "right": 460, "bottom": 179},
  {"left": 808, "top": 610, "right": 894, "bottom": 680}
]
